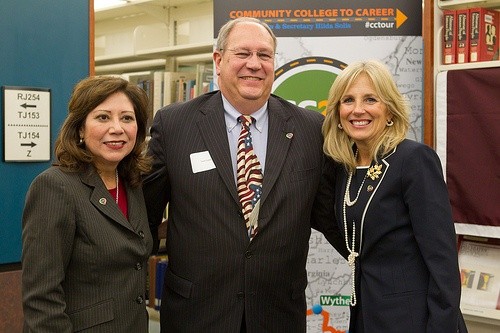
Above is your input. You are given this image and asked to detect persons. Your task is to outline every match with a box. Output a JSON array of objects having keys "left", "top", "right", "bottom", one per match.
[
  {"left": 142, "top": 16, "right": 348, "bottom": 333},
  {"left": 321, "top": 60, "right": 468, "bottom": 333},
  {"left": 21, "top": 75, "right": 168, "bottom": 333}
]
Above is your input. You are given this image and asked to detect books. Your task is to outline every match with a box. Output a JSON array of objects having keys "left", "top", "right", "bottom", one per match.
[{"left": 442, "top": 7, "right": 500, "bottom": 64}]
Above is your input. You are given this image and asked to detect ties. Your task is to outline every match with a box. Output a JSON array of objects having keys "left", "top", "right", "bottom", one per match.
[{"left": 234, "top": 114, "right": 264, "bottom": 239}]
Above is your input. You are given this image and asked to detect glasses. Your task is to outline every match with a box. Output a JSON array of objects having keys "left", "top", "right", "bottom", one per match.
[{"left": 218, "top": 48, "right": 275, "bottom": 60}]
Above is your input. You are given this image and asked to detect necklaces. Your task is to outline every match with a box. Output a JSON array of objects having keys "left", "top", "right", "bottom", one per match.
[
  {"left": 343, "top": 148, "right": 374, "bottom": 307},
  {"left": 116, "top": 169, "right": 119, "bottom": 204}
]
